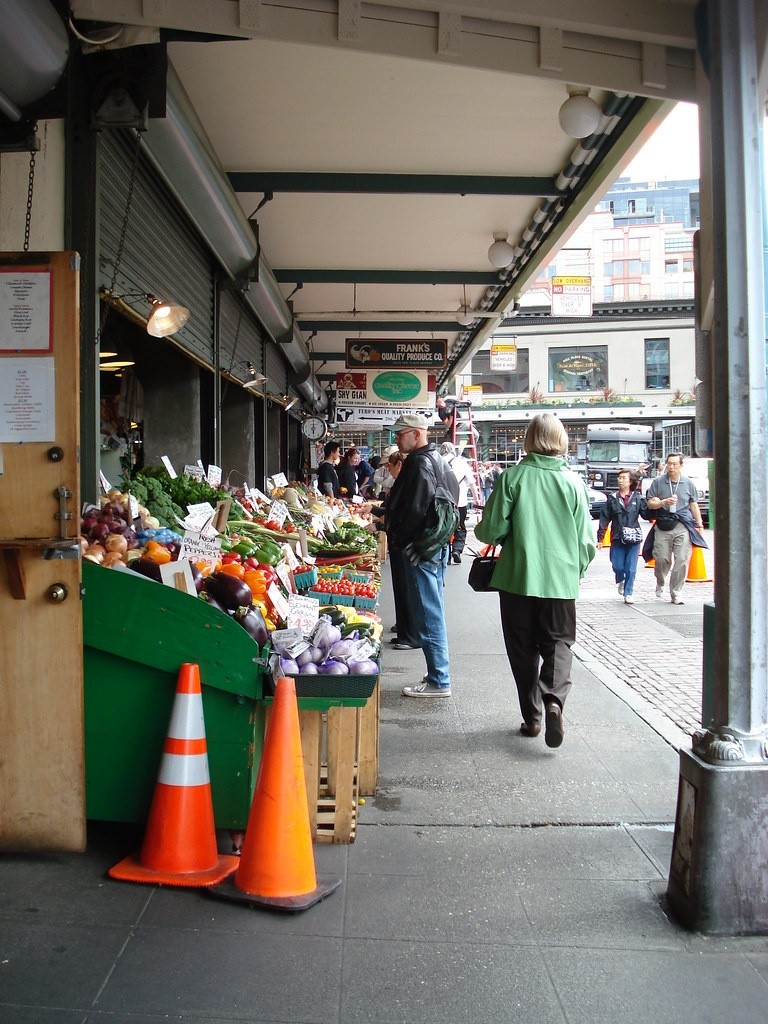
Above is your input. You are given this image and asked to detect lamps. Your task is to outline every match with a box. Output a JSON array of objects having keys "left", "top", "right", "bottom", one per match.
[
  {"left": 456, "top": 283, "right": 475, "bottom": 326},
  {"left": 558, "top": 84, "right": 600, "bottom": 139},
  {"left": 101, "top": 340, "right": 136, "bottom": 378},
  {"left": 100, "top": 284, "right": 190, "bottom": 339},
  {"left": 488, "top": 230, "right": 515, "bottom": 268}
]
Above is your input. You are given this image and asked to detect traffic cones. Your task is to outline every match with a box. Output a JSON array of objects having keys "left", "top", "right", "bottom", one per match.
[
  {"left": 107, "top": 664, "right": 238, "bottom": 888},
  {"left": 645, "top": 560, "right": 656, "bottom": 568},
  {"left": 208, "top": 676, "right": 342, "bottom": 915},
  {"left": 601, "top": 526, "right": 611, "bottom": 547},
  {"left": 685, "top": 545, "right": 713, "bottom": 582}
]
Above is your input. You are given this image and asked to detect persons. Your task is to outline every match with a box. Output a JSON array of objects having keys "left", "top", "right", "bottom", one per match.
[
  {"left": 355, "top": 452, "right": 422, "bottom": 649},
  {"left": 647, "top": 452, "right": 703, "bottom": 605},
  {"left": 356, "top": 461, "right": 371, "bottom": 497},
  {"left": 318, "top": 442, "right": 346, "bottom": 499},
  {"left": 374, "top": 467, "right": 394, "bottom": 490},
  {"left": 436, "top": 397, "right": 479, "bottom": 458},
  {"left": 439, "top": 442, "right": 480, "bottom": 564},
  {"left": 636, "top": 463, "right": 647, "bottom": 478},
  {"left": 479, "top": 463, "right": 503, "bottom": 504},
  {"left": 474, "top": 414, "right": 597, "bottom": 747},
  {"left": 385, "top": 414, "right": 451, "bottom": 698},
  {"left": 338, "top": 449, "right": 360, "bottom": 498},
  {"left": 597, "top": 468, "right": 661, "bottom": 603}
]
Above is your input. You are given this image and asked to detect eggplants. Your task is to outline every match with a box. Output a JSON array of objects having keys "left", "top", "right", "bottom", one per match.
[{"left": 128, "top": 557, "right": 269, "bottom": 649}]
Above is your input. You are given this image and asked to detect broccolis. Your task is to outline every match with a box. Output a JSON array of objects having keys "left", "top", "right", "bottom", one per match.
[{"left": 120, "top": 477, "right": 186, "bottom": 529}]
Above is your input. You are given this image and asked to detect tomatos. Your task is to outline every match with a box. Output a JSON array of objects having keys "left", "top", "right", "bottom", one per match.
[
  {"left": 217, "top": 551, "right": 378, "bottom": 599},
  {"left": 240, "top": 497, "right": 296, "bottom": 532}
]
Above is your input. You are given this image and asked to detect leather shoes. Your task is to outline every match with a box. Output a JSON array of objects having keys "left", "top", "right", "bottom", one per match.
[
  {"left": 545, "top": 701, "right": 564, "bottom": 748},
  {"left": 521, "top": 721, "right": 541, "bottom": 737}
]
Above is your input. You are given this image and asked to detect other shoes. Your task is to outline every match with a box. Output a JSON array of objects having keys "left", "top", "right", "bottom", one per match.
[
  {"left": 452, "top": 551, "right": 462, "bottom": 563},
  {"left": 394, "top": 643, "right": 422, "bottom": 650},
  {"left": 390, "top": 637, "right": 399, "bottom": 644},
  {"left": 618, "top": 579, "right": 625, "bottom": 595},
  {"left": 624, "top": 594, "right": 634, "bottom": 604},
  {"left": 447, "top": 561, "right": 451, "bottom": 565}
]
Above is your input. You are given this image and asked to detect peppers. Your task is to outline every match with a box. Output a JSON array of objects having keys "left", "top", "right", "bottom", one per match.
[{"left": 137, "top": 540, "right": 279, "bottom": 631}]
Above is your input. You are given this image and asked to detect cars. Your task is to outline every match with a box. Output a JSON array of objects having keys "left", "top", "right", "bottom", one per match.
[
  {"left": 660, "top": 457, "right": 713, "bottom": 522},
  {"left": 584, "top": 483, "right": 607, "bottom": 519}
]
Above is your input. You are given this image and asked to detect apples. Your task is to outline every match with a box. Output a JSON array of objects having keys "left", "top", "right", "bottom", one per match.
[{"left": 326, "top": 488, "right": 362, "bottom": 511}]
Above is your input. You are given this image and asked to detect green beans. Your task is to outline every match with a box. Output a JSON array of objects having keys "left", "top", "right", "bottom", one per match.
[{"left": 223, "top": 524, "right": 281, "bottom": 545}]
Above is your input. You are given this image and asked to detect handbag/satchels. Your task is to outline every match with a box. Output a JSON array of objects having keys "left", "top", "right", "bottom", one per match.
[
  {"left": 468, "top": 545, "right": 499, "bottom": 592},
  {"left": 656, "top": 513, "right": 679, "bottom": 531},
  {"left": 414, "top": 452, "right": 462, "bottom": 561},
  {"left": 620, "top": 526, "right": 642, "bottom": 544}
]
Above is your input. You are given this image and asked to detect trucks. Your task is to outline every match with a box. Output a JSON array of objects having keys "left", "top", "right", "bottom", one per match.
[{"left": 585, "top": 423, "right": 653, "bottom": 498}]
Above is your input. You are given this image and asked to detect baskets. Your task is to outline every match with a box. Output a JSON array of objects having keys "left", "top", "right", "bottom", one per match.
[{"left": 293, "top": 567, "right": 380, "bottom": 610}]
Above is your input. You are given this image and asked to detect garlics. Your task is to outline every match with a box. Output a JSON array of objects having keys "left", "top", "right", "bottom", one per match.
[{"left": 280, "top": 622, "right": 378, "bottom": 674}]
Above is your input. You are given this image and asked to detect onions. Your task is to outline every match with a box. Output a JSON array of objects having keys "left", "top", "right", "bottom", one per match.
[{"left": 78, "top": 490, "right": 160, "bottom": 569}]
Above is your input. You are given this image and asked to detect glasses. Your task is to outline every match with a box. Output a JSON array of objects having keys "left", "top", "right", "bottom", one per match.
[
  {"left": 616, "top": 477, "right": 630, "bottom": 481},
  {"left": 394, "top": 429, "right": 416, "bottom": 440}
]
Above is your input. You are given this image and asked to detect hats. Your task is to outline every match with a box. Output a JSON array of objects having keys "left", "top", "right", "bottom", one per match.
[{"left": 384, "top": 413, "right": 428, "bottom": 432}]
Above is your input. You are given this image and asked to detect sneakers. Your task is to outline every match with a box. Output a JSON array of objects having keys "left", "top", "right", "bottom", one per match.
[
  {"left": 422, "top": 674, "right": 428, "bottom": 682},
  {"left": 402, "top": 682, "right": 451, "bottom": 697},
  {"left": 655, "top": 582, "right": 666, "bottom": 597},
  {"left": 671, "top": 593, "right": 685, "bottom": 605}
]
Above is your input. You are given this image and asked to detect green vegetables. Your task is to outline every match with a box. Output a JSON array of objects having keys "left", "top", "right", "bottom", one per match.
[
  {"left": 312, "top": 520, "right": 381, "bottom": 553},
  {"left": 138, "top": 465, "right": 243, "bottom": 520}
]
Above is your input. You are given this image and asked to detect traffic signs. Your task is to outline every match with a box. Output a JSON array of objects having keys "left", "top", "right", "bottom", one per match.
[{"left": 333, "top": 408, "right": 436, "bottom": 427}]
